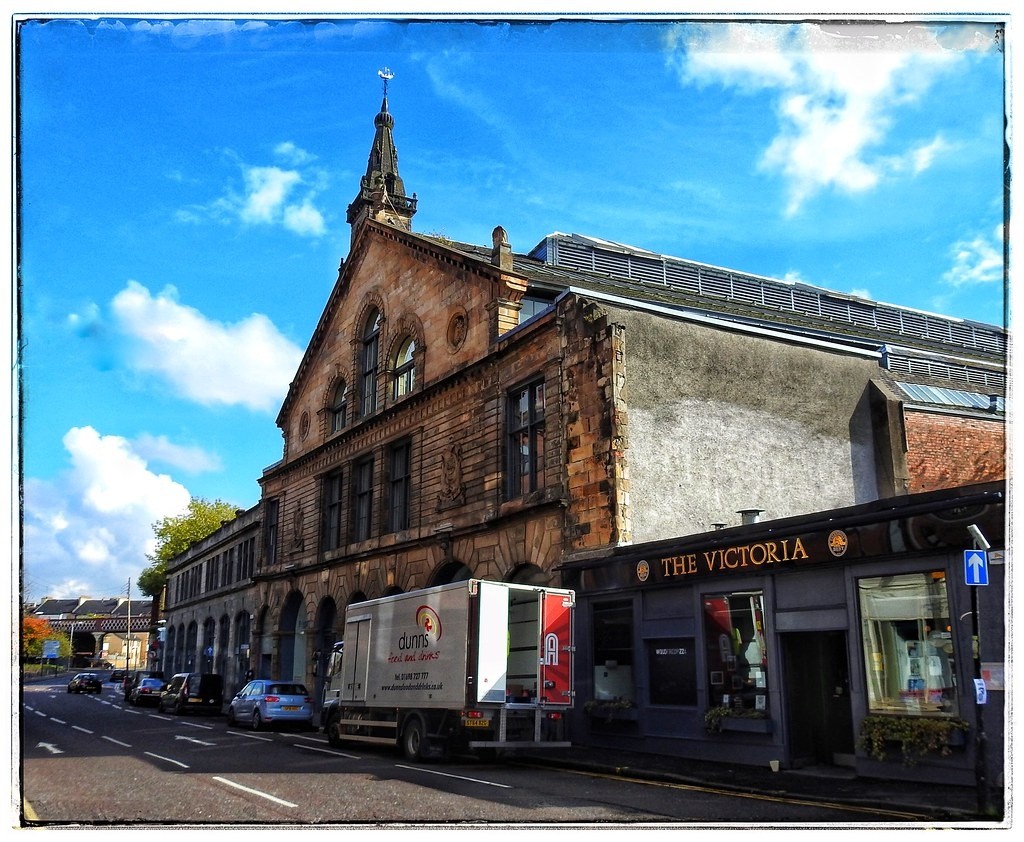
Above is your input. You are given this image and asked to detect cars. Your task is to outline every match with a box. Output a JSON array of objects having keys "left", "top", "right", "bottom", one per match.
[
  {"left": 130, "top": 678, "right": 166, "bottom": 708},
  {"left": 160, "top": 672, "right": 224, "bottom": 716},
  {"left": 125, "top": 671, "right": 165, "bottom": 700},
  {"left": 109, "top": 670, "right": 125, "bottom": 682},
  {"left": 67, "top": 672, "right": 102, "bottom": 695},
  {"left": 227, "top": 679, "right": 313, "bottom": 732}
]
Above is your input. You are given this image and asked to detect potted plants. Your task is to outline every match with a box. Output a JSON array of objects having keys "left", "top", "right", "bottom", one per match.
[
  {"left": 855, "top": 714, "right": 972, "bottom": 767},
  {"left": 705, "top": 706, "right": 774, "bottom": 737},
  {"left": 584, "top": 697, "right": 640, "bottom": 724}
]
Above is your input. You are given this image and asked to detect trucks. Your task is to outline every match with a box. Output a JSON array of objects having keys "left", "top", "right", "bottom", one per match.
[{"left": 310, "top": 578, "right": 577, "bottom": 765}]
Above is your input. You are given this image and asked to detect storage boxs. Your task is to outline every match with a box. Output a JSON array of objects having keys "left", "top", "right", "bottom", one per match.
[{"left": 507, "top": 684, "right": 524, "bottom": 696}]
